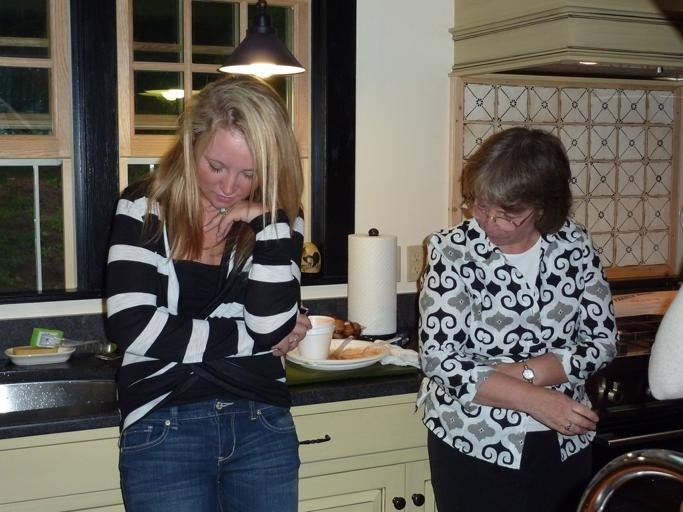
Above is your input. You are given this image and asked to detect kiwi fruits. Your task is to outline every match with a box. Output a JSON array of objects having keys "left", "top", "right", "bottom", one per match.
[{"left": 333, "top": 318, "right": 361, "bottom": 339}]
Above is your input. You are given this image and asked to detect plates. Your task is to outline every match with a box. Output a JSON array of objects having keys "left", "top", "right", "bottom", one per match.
[
  {"left": 285, "top": 338, "right": 390, "bottom": 371},
  {"left": 4, "top": 346, "right": 77, "bottom": 365}
]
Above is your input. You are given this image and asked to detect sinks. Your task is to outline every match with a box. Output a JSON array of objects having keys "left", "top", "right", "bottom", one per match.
[{"left": 0, "top": 373, "right": 118, "bottom": 417}]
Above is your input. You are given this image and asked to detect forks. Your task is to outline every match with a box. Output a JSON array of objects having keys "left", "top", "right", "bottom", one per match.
[{"left": 342, "top": 336, "right": 403, "bottom": 358}]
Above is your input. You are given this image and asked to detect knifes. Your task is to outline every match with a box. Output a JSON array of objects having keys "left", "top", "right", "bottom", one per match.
[{"left": 329, "top": 335, "right": 354, "bottom": 358}]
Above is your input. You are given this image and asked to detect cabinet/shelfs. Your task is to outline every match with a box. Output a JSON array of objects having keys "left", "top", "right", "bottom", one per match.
[
  {"left": 0, "top": 433, "right": 120, "bottom": 503},
  {"left": 290, "top": 400, "right": 440, "bottom": 512}
]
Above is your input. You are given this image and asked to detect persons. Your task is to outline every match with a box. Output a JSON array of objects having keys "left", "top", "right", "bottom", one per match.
[
  {"left": 101, "top": 72, "right": 314, "bottom": 511},
  {"left": 414, "top": 124, "right": 621, "bottom": 512}
]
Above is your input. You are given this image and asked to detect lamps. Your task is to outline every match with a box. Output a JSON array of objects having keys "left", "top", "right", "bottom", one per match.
[{"left": 215, "top": 1, "right": 309, "bottom": 81}]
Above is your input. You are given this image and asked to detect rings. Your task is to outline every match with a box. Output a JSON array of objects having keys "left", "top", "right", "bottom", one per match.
[
  {"left": 216, "top": 207, "right": 229, "bottom": 215},
  {"left": 563, "top": 421, "right": 572, "bottom": 430},
  {"left": 288, "top": 333, "right": 298, "bottom": 343}
]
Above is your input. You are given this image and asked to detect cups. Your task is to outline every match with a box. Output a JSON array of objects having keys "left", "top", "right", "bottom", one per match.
[{"left": 299, "top": 314, "right": 336, "bottom": 360}]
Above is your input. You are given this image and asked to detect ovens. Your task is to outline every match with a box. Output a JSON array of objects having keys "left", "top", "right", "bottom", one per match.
[{"left": 584, "top": 404, "right": 683, "bottom": 511}]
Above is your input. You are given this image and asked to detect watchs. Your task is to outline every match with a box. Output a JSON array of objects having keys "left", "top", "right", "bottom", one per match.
[{"left": 520, "top": 361, "right": 535, "bottom": 383}]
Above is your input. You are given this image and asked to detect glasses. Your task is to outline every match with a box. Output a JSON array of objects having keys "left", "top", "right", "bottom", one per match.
[{"left": 461, "top": 198, "right": 537, "bottom": 231}]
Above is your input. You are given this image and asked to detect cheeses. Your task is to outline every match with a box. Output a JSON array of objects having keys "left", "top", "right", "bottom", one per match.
[{"left": 14, "top": 346, "right": 58, "bottom": 356}]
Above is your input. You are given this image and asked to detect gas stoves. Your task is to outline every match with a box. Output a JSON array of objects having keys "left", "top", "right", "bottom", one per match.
[{"left": 584, "top": 314, "right": 664, "bottom": 409}]
